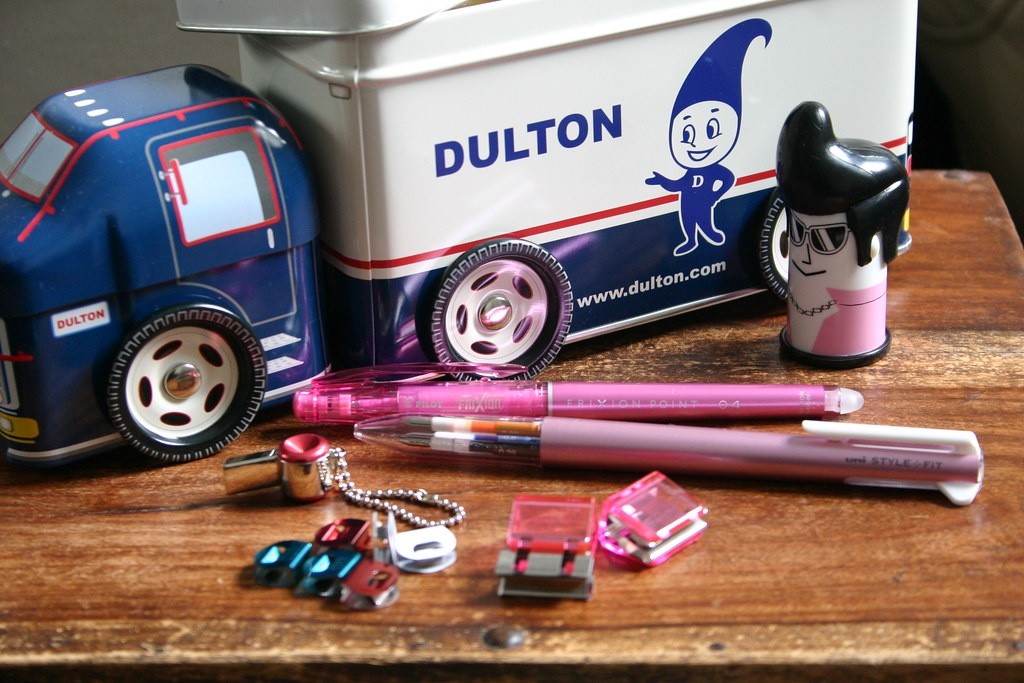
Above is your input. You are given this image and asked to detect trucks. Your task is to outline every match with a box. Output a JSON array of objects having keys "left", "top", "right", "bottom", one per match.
[{"left": 1, "top": 0, "right": 919, "bottom": 467}]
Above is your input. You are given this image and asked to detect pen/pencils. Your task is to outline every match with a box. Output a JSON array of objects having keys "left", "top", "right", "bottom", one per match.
[
  {"left": 294, "top": 360, "right": 865, "bottom": 426},
  {"left": 354, "top": 411, "right": 984, "bottom": 506}
]
